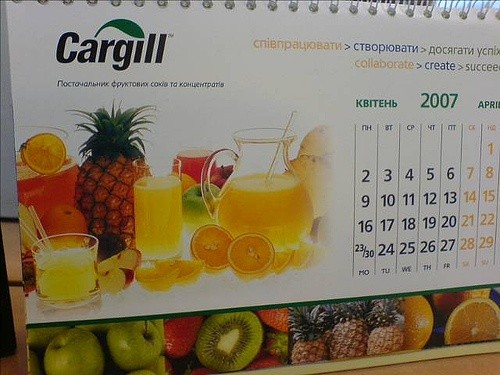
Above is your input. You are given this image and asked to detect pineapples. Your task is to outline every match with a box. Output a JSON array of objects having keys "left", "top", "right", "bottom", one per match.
[
  {"left": 288, "top": 296, "right": 403, "bottom": 366},
  {"left": 71, "top": 103, "right": 157, "bottom": 249}
]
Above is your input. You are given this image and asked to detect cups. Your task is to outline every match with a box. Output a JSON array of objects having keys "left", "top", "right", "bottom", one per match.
[
  {"left": 131, "top": 156, "right": 182, "bottom": 266},
  {"left": 30, "top": 233, "right": 99, "bottom": 307}
]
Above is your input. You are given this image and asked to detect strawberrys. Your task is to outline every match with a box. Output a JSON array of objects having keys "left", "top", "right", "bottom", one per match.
[{"left": 164, "top": 305, "right": 291, "bottom": 375}]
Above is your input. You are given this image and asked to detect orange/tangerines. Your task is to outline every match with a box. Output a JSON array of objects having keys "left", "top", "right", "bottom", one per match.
[
  {"left": 20, "top": 133, "right": 67, "bottom": 175},
  {"left": 133, "top": 225, "right": 311, "bottom": 289},
  {"left": 395, "top": 289, "right": 500, "bottom": 350},
  {"left": 37, "top": 205, "right": 87, "bottom": 240}
]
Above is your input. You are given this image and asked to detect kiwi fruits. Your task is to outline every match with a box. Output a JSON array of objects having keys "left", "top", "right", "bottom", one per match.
[{"left": 195, "top": 311, "right": 264, "bottom": 373}]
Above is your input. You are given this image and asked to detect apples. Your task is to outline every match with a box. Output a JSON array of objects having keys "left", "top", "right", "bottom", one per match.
[
  {"left": 93, "top": 246, "right": 140, "bottom": 294},
  {"left": 181, "top": 164, "right": 237, "bottom": 227},
  {"left": 26, "top": 318, "right": 167, "bottom": 375}
]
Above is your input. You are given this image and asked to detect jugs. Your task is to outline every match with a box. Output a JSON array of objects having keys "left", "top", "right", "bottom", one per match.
[{"left": 199, "top": 126, "right": 314, "bottom": 253}]
